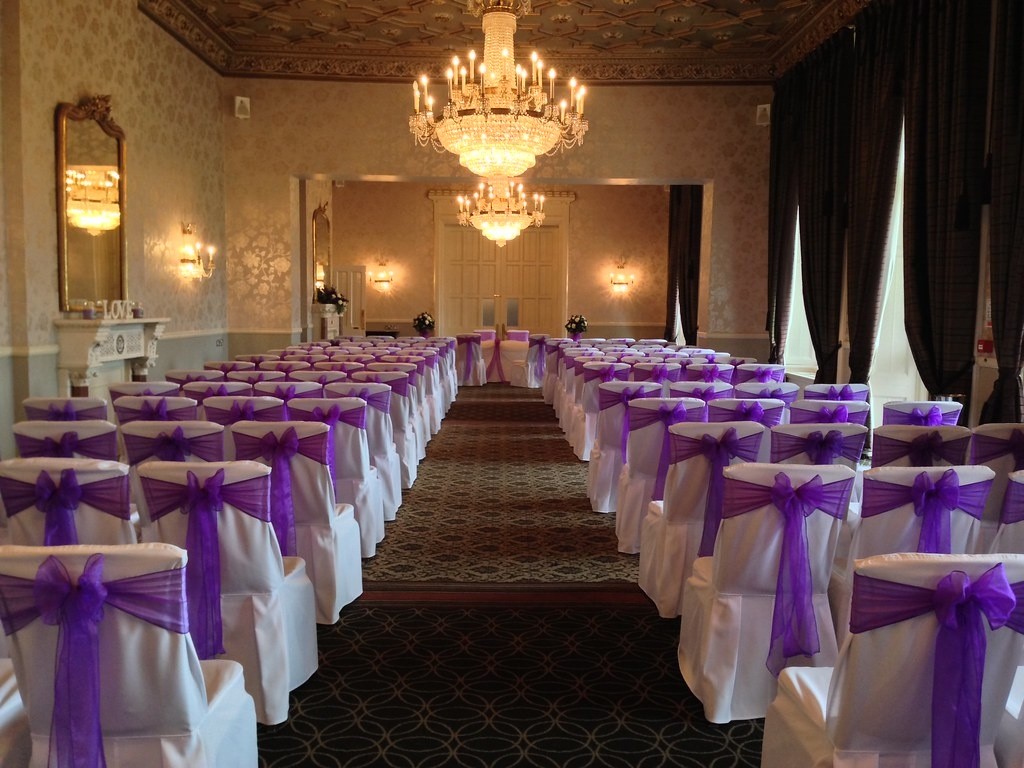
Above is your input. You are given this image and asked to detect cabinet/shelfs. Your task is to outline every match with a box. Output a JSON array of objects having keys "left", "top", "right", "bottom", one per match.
[{"left": 312, "top": 304, "right": 339, "bottom": 341}]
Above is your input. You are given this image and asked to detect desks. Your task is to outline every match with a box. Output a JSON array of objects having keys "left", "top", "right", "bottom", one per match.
[{"left": 481, "top": 340, "right": 530, "bottom": 382}]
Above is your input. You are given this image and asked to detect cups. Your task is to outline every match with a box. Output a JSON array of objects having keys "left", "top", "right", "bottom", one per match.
[{"left": 935, "top": 396, "right": 952, "bottom": 401}]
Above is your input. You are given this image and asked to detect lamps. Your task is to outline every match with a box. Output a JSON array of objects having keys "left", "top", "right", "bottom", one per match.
[
  {"left": 177, "top": 219, "right": 215, "bottom": 281},
  {"left": 608, "top": 255, "right": 635, "bottom": 293},
  {"left": 65, "top": 166, "right": 120, "bottom": 236},
  {"left": 367, "top": 260, "right": 393, "bottom": 289},
  {"left": 409, "top": 0, "right": 589, "bottom": 247}
]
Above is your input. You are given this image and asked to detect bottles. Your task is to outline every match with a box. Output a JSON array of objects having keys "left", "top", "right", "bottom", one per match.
[
  {"left": 978, "top": 321, "right": 994, "bottom": 358},
  {"left": 63, "top": 299, "right": 144, "bottom": 319}
]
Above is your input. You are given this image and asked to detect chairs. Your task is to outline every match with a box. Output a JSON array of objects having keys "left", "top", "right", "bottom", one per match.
[
  {"left": 0, "top": 334, "right": 458, "bottom": 768},
  {"left": 510, "top": 334, "right": 550, "bottom": 388},
  {"left": 542, "top": 339, "right": 1024, "bottom": 767},
  {"left": 455, "top": 333, "right": 488, "bottom": 387},
  {"left": 472, "top": 330, "right": 496, "bottom": 341},
  {"left": 506, "top": 330, "right": 529, "bottom": 342}
]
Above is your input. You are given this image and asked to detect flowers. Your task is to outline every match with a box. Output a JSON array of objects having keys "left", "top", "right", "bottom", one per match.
[
  {"left": 317, "top": 284, "right": 349, "bottom": 316},
  {"left": 563, "top": 315, "right": 587, "bottom": 332},
  {"left": 413, "top": 312, "right": 435, "bottom": 332}
]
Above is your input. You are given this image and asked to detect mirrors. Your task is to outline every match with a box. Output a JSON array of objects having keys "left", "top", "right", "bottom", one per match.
[
  {"left": 313, "top": 201, "right": 331, "bottom": 302},
  {"left": 55, "top": 93, "right": 127, "bottom": 313}
]
